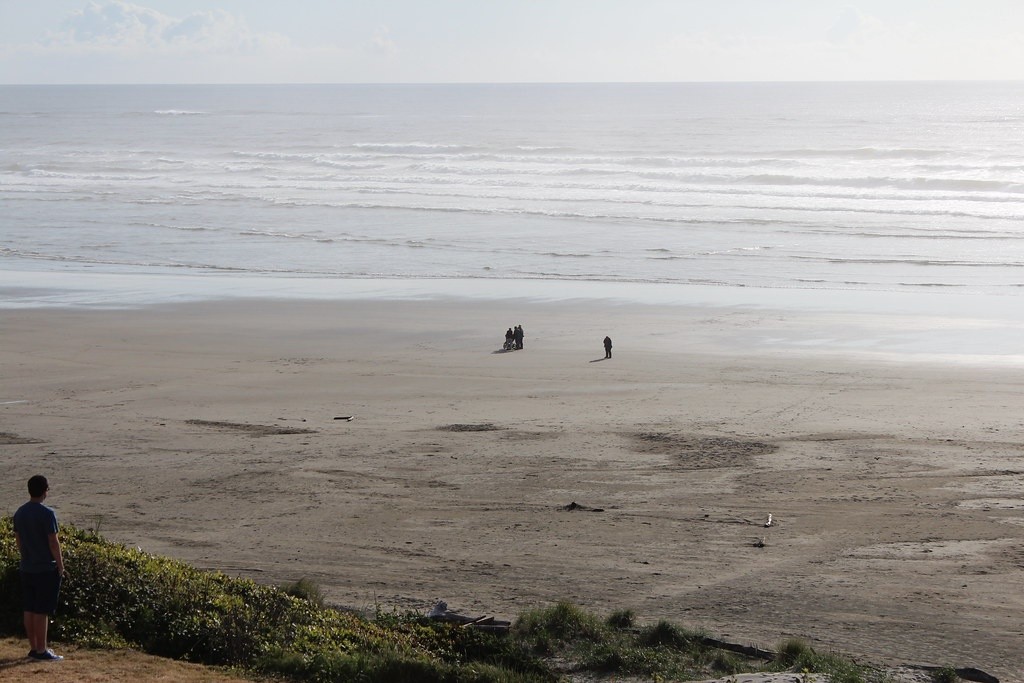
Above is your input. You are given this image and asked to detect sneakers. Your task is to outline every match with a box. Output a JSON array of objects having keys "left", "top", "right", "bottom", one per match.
[{"left": 28, "top": 649, "right": 63, "bottom": 662}]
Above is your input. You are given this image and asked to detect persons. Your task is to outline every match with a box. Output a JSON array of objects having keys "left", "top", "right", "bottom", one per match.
[
  {"left": 505, "top": 324, "right": 524, "bottom": 350},
  {"left": 603, "top": 336, "right": 612, "bottom": 358},
  {"left": 11, "top": 474, "right": 65, "bottom": 660}
]
